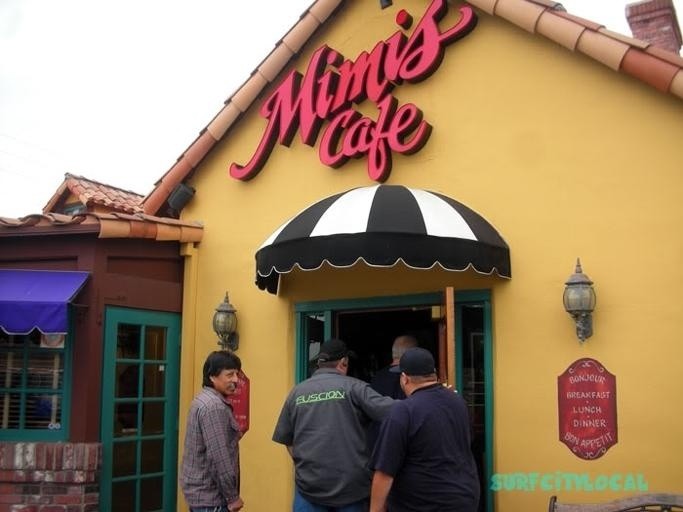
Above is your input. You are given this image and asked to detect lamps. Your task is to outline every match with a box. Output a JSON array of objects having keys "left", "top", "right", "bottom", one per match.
[
  {"left": 213, "top": 291, "right": 239, "bottom": 351},
  {"left": 561, "top": 257, "right": 595, "bottom": 344}
]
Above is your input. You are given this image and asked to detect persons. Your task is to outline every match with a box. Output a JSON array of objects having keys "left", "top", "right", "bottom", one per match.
[
  {"left": 178, "top": 350, "right": 244, "bottom": 512},
  {"left": 272, "top": 338, "right": 452, "bottom": 511},
  {"left": 376, "top": 333, "right": 417, "bottom": 399},
  {"left": 369, "top": 346, "right": 480, "bottom": 511}
]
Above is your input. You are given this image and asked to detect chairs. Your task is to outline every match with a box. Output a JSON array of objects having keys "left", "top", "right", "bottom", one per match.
[{"left": 548, "top": 493, "right": 683, "bottom": 512}]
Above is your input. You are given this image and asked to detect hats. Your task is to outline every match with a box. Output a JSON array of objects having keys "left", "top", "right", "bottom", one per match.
[
  {"left": 389, "top": 348, "right": 434, "bottom": 374},
  {"left": 318, "top": 339, "right": 353, "bottom": 361}
]
